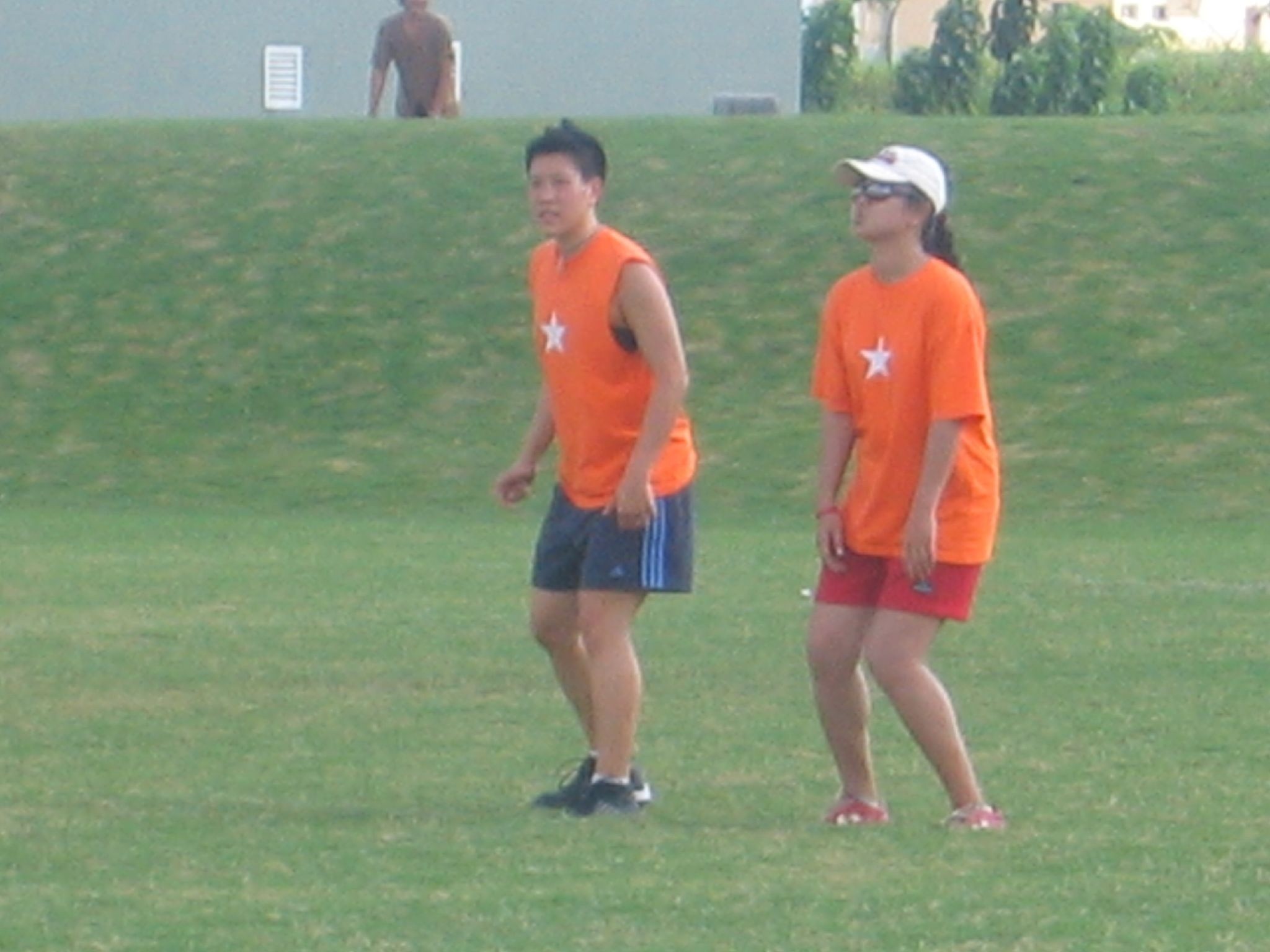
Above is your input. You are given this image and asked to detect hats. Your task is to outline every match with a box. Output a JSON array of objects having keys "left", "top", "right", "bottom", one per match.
[{"left": 835, "top": 145, "right": 947, "bottom": 217}]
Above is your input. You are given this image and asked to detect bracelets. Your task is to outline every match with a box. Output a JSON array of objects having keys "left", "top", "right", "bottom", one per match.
[{"left": 816, "top": 505, "right": 835, "bottom": 518}]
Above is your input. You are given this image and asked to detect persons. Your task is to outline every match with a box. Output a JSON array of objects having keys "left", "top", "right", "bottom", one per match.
[
  {"left": 496, "top": 116, "right": 699, "bottom": 814},
  {"left": 805, "top": 142, "right": 1002, "bottom": 833},
  {"left": 365, "top": 0, "right": 459, "bottom": 118}
]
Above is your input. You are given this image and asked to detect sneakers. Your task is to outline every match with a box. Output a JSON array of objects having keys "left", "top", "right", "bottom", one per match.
[
  {"left": 824, "top": 793, "right": 889, "bottom": 828},
  {"left": 528, "top": 757, "right": 657, "bottom": 810},
  {"left": 563, "top": 770, "right": 640, "bottom": 821},
  {"left": 942, "top": 804, "right": 1007, "bottom": 833}
]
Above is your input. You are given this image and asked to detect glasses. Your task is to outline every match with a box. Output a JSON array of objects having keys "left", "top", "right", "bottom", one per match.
[{"left": 850, "top": 181, "right": 918, "bottom": 201}]
